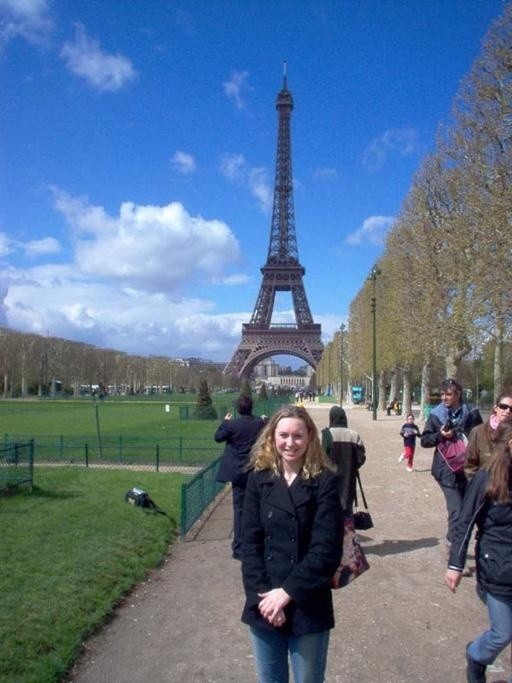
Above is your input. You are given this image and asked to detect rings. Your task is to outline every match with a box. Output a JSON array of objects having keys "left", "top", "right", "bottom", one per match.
[{"left": 277, "top": 616, "right": 282, "bottom": 622}]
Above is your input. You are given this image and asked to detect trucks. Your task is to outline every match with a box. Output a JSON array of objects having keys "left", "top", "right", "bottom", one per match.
[{"left": 351, "top": 386, "right": 365, "bottom": 404}]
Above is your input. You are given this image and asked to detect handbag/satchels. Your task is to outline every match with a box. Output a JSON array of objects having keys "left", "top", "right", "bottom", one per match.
[
  {"left": 352, "top": 511, "right": 375, "bottom": 530},
  {"left": 330, "top": 528, "right": 369, "bottom": 589},
  {"left": 437, "top": 433, "right": 471, "bottom": 473},
  {"left": 125, "top": 488, "right": 148, "bottom": 507}
]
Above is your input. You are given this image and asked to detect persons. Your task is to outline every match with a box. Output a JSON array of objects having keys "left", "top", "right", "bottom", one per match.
[
  {"left": 214, "top": 394, "right": 270, "bottom": 559},
  {"left": 420, "top": 378, "right": 485, "bottom": 577},
  {"left": 443, "top": 429, "right": 512, "bottom": 682},
  {"left": 462, "top": 392, "right": 511, "bottom": 484},
  {"left": 317, "top": 405, "right": 369, "bottom": 530},
  {"left": 397, "top": 413, "right": 421, "bottom": 472},
  {"left": 295, "top": 390, "right": 315, "bottom": 402},
  {"left": 240, "top": 404, "right": 344, "bottom": 682}
]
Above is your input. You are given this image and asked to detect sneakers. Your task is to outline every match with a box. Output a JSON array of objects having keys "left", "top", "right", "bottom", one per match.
[
  {"left": 398, "top": 453, "right": 413, "bottom": 472},
  {"left": 465, "top": 639, "right": 486, "bottom": 683}
]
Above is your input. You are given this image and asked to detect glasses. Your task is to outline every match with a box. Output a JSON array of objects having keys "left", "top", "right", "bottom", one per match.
[{"left": 498, "top": 402, "right": 512, "bottom": 411}]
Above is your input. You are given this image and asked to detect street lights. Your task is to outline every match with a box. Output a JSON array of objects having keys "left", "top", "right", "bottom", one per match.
[
  {"left": 327, "top": 340, "right": 334, "bottom": 397},
  {"left": 367, "top": 263, "right": 383, "bottom": 421},
  {"left": 339, "top": 321, "right": 347, "bottom": 408}
]
